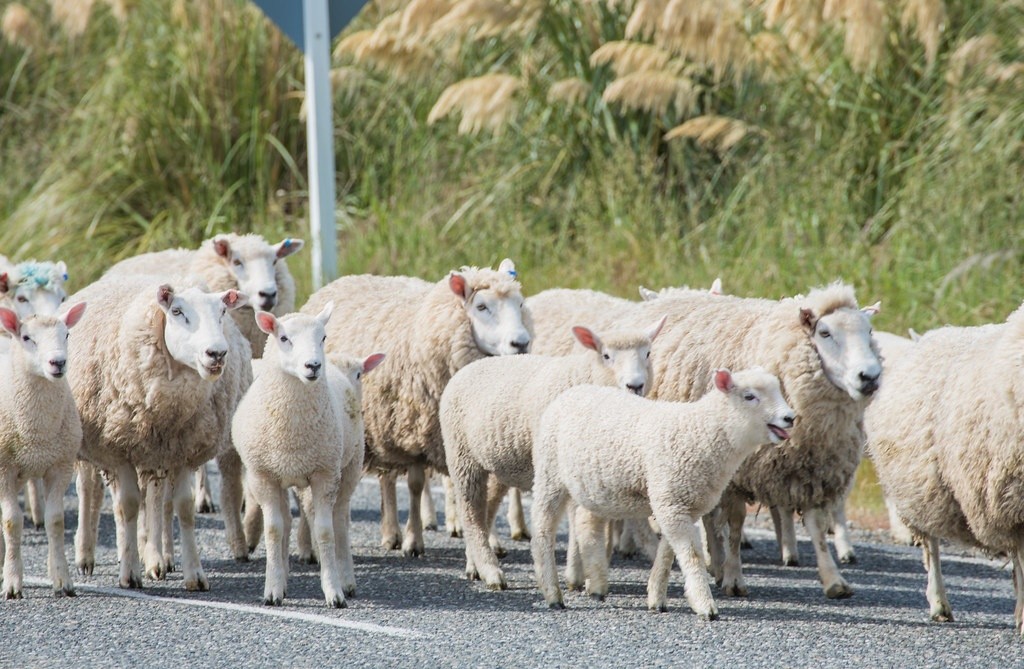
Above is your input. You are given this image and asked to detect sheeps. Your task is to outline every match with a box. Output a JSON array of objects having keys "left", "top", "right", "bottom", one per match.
[{"left": 0, "top": 226, "right": 1022, "bottom": 633}]
